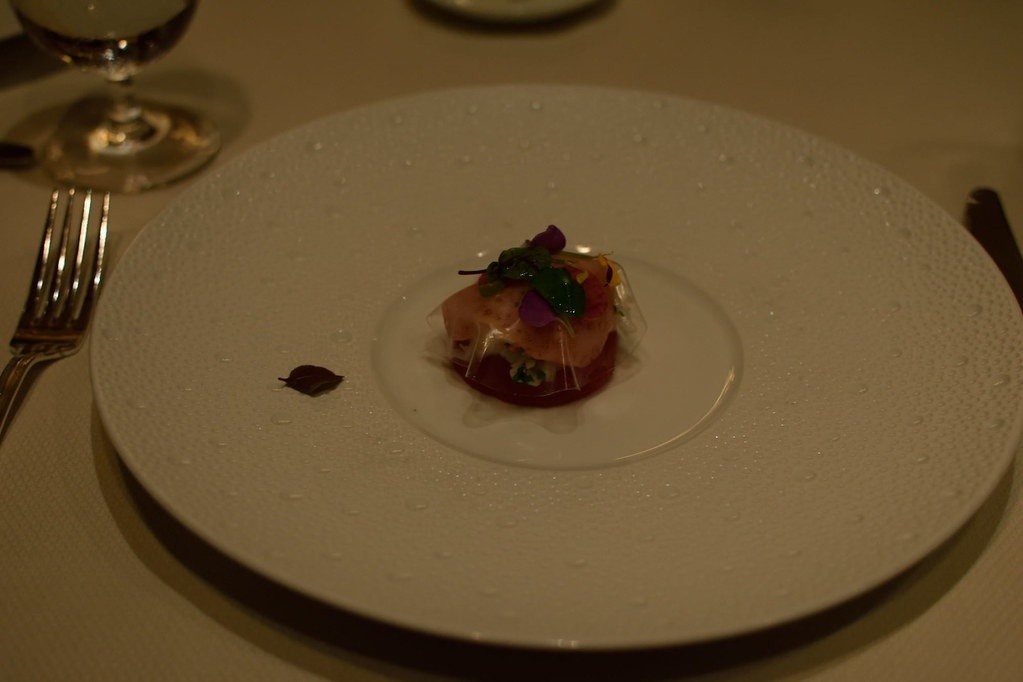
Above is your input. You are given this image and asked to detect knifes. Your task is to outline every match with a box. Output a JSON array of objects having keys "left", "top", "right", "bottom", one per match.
[{"left": 964, "top": 190, "right": 1022, "bottom": 309}]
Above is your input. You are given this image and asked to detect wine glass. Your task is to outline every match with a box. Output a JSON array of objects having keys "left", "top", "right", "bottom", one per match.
[{"left": 9, "top": 0, "right": 226, "bottom": 199}]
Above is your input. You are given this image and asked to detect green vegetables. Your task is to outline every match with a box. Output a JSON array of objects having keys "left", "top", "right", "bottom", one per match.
[{"left": 456, "top": 240, "right": 585, "bottom": 319}]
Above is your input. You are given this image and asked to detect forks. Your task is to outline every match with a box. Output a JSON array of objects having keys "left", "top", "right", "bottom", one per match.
[{"left": 1, "top": 186, "right": 112, "bottom": 442}]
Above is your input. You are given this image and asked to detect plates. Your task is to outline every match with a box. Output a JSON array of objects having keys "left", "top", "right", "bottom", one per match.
[{"left": 86, "top": 80, "right": 1023, "bottom": 654}]
